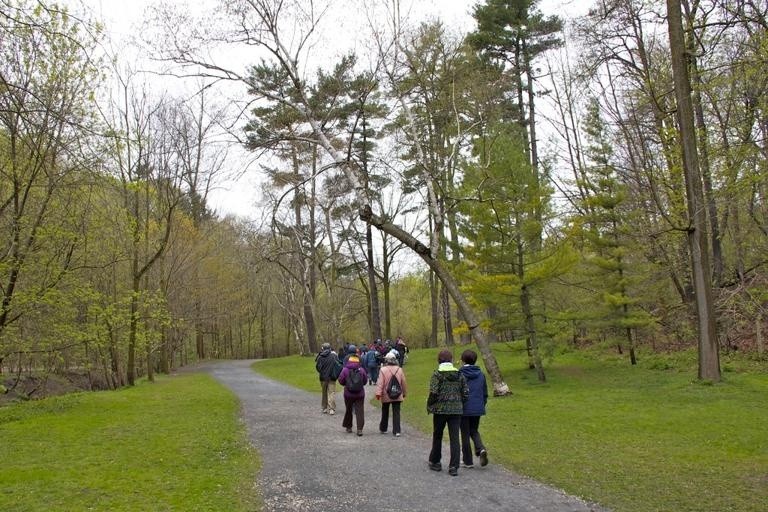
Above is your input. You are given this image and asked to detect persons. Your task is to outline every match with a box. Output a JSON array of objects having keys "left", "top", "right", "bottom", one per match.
[
  {"left": 375, "top": 352, "right": 407, "bottom": 436},
  {"left": 425, "top": 349, "right": 470, "bottom": 476},
  {"left": 314, "top": 335, "right": 410, "bottom": 386},
  {"left": 316, "top": 342, "right": 341, "bottom": 413},
  {"left": 338, "top": 354, "right": 369, "bottom": 435},
  {"left": 458, "top": 350, "right": 490, "bottom": 468}
]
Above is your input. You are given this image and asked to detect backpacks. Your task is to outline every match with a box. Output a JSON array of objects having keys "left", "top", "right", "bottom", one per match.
[
  {"left": 388, "top": 368, "right": 402, "bottom": 399},
  {"left": 347, "top": 368, "right": 363, "bottom": 393}
]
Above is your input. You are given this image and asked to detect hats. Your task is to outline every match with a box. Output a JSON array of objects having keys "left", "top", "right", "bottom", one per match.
[{"left": 383, "top": 353, "right": 396, "bottom": 359}]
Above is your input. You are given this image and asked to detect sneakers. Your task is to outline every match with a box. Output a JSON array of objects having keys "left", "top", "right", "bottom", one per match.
[{"left": 430, "top": 449, "right": 488, "bottom": 475}]
